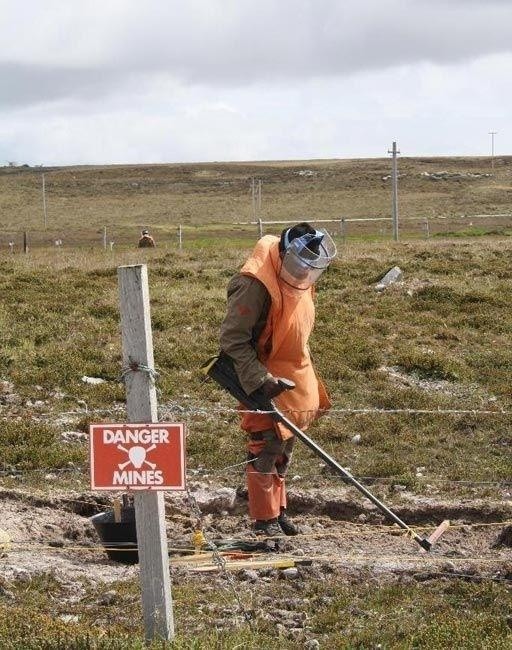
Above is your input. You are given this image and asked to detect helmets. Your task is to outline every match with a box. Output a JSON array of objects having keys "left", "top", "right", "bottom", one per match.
[{"left": 279, "top": 230, "right": 339, "bottom": 290}]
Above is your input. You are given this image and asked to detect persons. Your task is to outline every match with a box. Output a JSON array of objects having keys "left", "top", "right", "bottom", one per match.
[
  {"left": 136, "top": 229, "right": 157, "bottom": 248},
  {"left": 219, "top": 220, "right": 332, "bottom": 546}
]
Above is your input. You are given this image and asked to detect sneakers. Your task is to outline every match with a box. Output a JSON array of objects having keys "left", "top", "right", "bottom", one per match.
[
  {"left": 279, "top": 512, "right": 312, "bottom": 534},
  {"left": 252, "top": 520, "right": 287, "bottom": 538}
]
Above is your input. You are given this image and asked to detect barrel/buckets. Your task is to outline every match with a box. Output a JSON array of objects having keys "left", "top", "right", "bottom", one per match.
[{"left": 92, "top": 508, "right": 138, "bottom": 565}]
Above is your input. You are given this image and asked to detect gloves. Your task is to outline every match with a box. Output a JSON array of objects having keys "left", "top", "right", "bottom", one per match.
[{"left": 264, "top": 375, "right": 287, "bottom": 399}]
